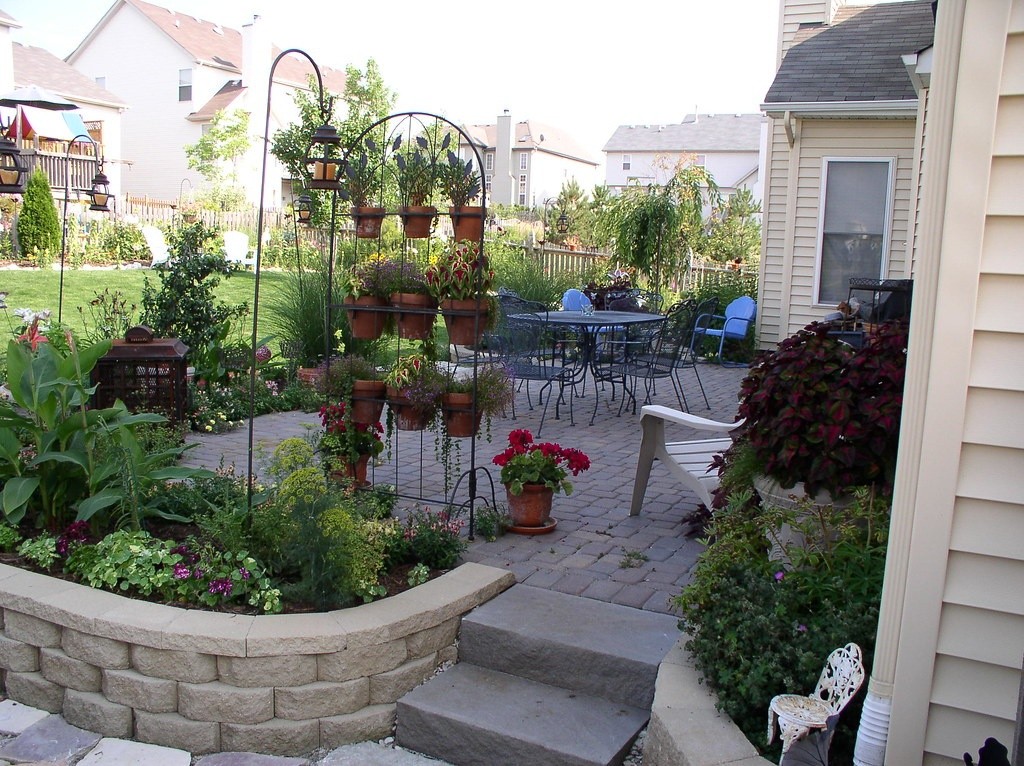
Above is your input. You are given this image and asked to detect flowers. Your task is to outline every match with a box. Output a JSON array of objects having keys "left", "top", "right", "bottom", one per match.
[
  {"left": 704, "top": 320, "right": 910, "bottom": 513},
  {"left": 316, "top": 354, "right": 515, "bottom": 462},
  {"left": 337, "top": 239, "right": 494, "bottom": 304},
  {"left": 492, "top": 429, "right": 591, "bottom": 496}
]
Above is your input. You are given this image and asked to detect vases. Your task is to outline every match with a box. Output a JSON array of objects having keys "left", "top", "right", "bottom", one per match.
[
  {"left": 441, "top": 300, "right": 488, "bottom": 344},
  {"left": 298, "top": 368, "right": 318, "bottom": 384},
  {"left": 336, "top": 454, "right": 373, "bottom": 490},
  {"left": 385, "top": 387, "right": 427, "bottom": 430},
  {"left": 752, "top": 471, "right": 870, "bottom": 572},
  {"left": 501, "top": 482, "right": 557, "bottom": 534},
  {"left": 343, "top": 296, "right": 387, "bottom": 338},
  {"left": 350, "top": 380, "right": 385, "bottom": 423},
  {"left": 390, "top": 293, "right": 439, "bottom": 338},
  {"left": 440, "top": 392, "right": 484, "bottom": 437}
]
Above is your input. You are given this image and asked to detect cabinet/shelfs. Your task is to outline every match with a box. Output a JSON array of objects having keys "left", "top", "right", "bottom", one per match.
[{"left": 314, "top": 112, "right": 500, "bottom": 541}]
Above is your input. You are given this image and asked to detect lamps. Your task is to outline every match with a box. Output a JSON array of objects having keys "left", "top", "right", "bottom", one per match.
[
  {"left": 543, "top": 197, "right": 569, "bottom": 247},
  {"left": 289, "top": 168, "right": 313, "bottom": 297},
  {"left": 585, "top": 235, "right": 598, "bottom": 261},
  {"left": 0, "top": 125, "right": 29, "bottom": 196},
  {"left": 244, "top": 45, "right": 347, "bottom": 535},
  {"left": 59, "top": 135, "right": 115, "bottom": 322}
]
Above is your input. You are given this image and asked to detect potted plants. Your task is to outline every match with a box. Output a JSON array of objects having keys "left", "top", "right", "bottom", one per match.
[
  {"left": 386, "top": 133, "right": 451, "bottom": 238},
  {"left": 181, "top": 209, "right": 197, "bottom": 222},
  {"left": 438, "top": 149, "right": 487, "bottom": 244},
  {"left": 342, "top": 139, "right": 386, "bottom": 238}
]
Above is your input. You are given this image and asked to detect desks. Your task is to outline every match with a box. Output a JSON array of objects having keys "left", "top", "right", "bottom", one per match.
[{"left": 507, "top": 310, "right": 668, "bottom": 405}]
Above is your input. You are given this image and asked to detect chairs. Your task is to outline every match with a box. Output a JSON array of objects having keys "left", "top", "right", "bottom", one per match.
[
  {"left": 449, "top": 285, "right": 720, "bottom": 439},
  {"left": 767, "top": 642, "right": 865, "bottom": 766},
  {"left": 690, "top": 296, "right": 757, "bottom": 368},
  {"left": 628, "top": 405, "right": 746, "bottom": 543}
]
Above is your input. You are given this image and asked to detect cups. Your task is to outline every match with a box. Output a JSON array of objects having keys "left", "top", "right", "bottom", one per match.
[{"left": 581, "top": 305, "right": 595, "bottom": 316}]
